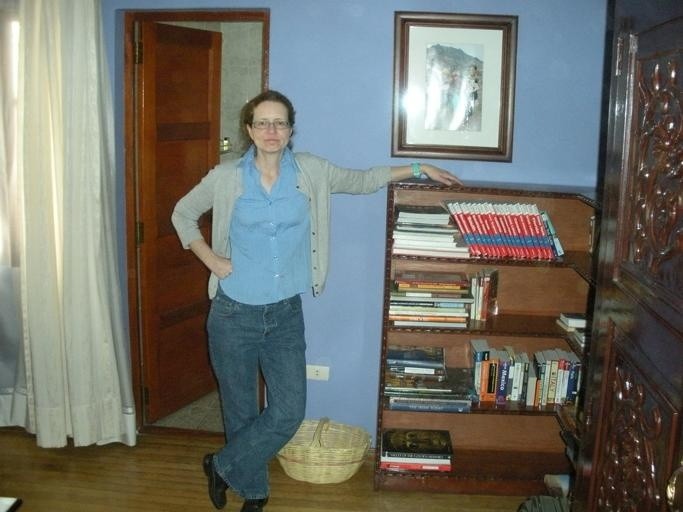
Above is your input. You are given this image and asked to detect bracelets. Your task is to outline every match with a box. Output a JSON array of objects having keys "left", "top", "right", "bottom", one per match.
[{"left": 411, "top": 161, "right": 421, "bottom": 179}]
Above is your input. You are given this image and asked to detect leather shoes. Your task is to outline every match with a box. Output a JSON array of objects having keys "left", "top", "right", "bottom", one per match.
[
  {"left": 240, "top": 497, "right": 268, "bottom": 512},
  {"left": 203, "top": 453, "right": 229, "bottom": 509}
]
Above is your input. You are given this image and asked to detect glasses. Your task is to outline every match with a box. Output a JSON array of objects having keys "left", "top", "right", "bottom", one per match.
[{"left": 252, "top": 120, "right": 290, "bottom": 129}]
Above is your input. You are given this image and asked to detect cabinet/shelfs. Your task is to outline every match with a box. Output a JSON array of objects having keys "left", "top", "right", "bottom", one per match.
[{"left": 372, "top": 181, "right": 604, "bottom": 494}]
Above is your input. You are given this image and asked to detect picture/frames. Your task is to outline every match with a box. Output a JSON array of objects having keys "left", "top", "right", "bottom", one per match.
[{"left": 393, "top": 8, "right": 519, "bottom": 163}]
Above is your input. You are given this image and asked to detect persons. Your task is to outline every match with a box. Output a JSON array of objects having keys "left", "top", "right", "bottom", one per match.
[{"left": 170, "top": 90, "right": 464, "bottom": 512}]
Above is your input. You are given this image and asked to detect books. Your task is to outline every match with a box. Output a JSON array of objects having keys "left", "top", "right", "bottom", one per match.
[
  {"left": 557, "top": 312, "right": 586, "bottom": 348},
  {"left": 390, "top": 202, "right": 564, "bottom": 260},
  {"left": 381, "top": 429, "right": 453, "bottom": 472},
  {"left": 470, "top": 339, "right": 582, "bottom": 406},
  {"left": 383, "top": 345, "right": 479, "bottom": 412},
  {"left": 389, "top": 269, "right": 498, "bottom": 327}
]
